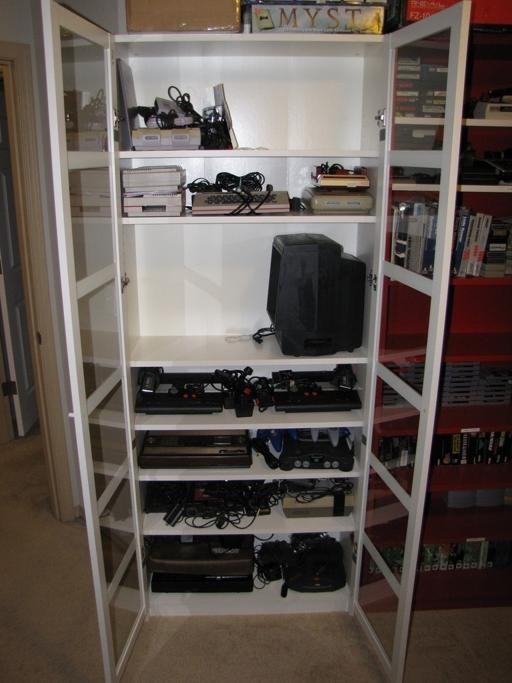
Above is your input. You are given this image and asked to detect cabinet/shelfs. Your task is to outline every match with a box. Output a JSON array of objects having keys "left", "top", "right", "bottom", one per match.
[
  {"left": 36, "top": 0, "right": 477, "bottom": 682},
  {"left": 358, "top": 31, "right": 512, "bottom": 616}
]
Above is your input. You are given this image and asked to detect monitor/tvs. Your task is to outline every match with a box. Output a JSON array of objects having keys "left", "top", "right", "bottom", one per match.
[{"left": 266, "top": 232, "right": 366, "bottom": 356}]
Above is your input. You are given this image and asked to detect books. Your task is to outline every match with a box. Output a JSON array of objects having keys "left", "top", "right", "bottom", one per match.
[
  {"left": 368, "top": 539, "right": 494, "bottom": 576},
  {"left": 378, "top": 432, "right": 512, "bottom": 470},
  {"left": 397, "top": 50, "right": 468, "bottom": 117},
  {"left": 390, "top": 196, "right": 512, "bottom": 281}
]
